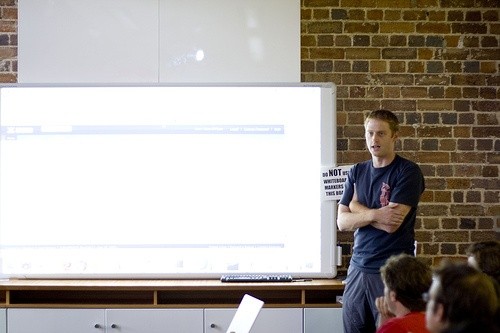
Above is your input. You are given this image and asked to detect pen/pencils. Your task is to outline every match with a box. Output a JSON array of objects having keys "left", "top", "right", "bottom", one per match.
[{"left": 292, "top": 279, "right": 312, "bottom": 282}]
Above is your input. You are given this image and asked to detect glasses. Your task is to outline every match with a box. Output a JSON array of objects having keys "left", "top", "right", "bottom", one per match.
[{"left": 422, "top": 293, "right": 448, "bottom": 302}]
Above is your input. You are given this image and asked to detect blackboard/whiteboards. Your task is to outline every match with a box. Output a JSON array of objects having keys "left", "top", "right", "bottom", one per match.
[{"left": 0, "top": 81, "right": 336, "bottom": 279}]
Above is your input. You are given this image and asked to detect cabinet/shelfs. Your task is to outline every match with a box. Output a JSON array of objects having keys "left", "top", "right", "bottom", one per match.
[{"left": 1, "top": 276, "right": 345, "bottom": 333}]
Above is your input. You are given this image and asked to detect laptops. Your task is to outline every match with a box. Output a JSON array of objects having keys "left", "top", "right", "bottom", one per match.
[{"left": 226, "top": 294, "right": 264, "bottom": 333}]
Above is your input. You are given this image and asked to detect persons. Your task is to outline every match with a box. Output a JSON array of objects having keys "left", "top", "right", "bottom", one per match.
[
  {"left": 337, "top": 109, "right": 426, "bottom": 333},
  {"left": 375, "top": 240, "right": 500, "bottom": 333}
]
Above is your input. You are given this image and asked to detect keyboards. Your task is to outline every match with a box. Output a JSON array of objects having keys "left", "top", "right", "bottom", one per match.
[{"left": 221, "top": 274, "right": 292, "bottom": 282}]
